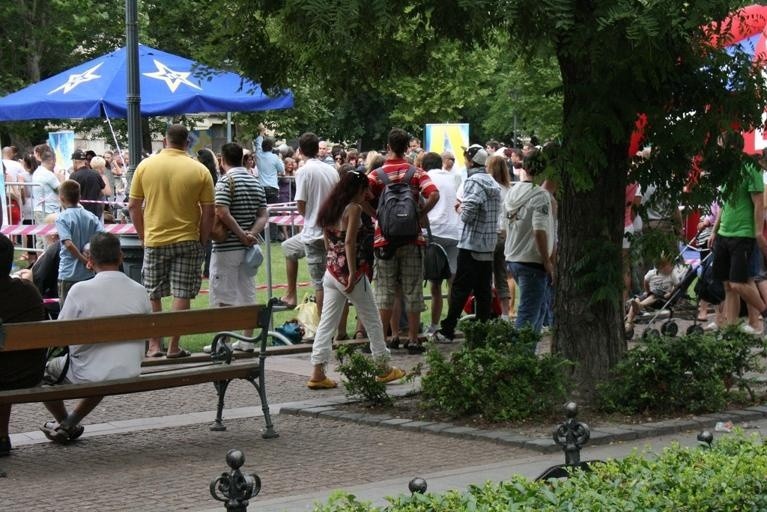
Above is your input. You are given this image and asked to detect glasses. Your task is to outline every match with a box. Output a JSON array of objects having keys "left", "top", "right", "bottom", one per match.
[{"left": 335, "top": 158, "right": 342, "bottom": 161}]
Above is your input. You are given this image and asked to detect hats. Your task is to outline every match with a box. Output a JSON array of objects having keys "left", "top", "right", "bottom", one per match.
[
  {"left": 460, "top": 143, "right": 488, "bottom": 167},
  {"left": 244, "top": 244, "right": 264, "bottom": 277},
  {"left": 71, "top": 149, "right": 86, "bottom": 160}
]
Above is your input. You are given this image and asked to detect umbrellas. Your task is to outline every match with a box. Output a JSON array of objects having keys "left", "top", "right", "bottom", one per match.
[{"left": 0, "top": 44, "right": 294, "bottom": 119}]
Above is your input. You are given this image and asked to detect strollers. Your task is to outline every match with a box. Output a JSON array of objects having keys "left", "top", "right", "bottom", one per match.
[{"left": 620, "top": 223, "right": 718, "bottom": 339}]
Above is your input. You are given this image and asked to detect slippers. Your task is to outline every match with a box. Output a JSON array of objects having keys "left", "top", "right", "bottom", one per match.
[
  {"left": 166, "top": 347, "right": 191, "bottom": 358},
  {"left": 306, "top": 377, "right": 338, "bottom": 389},
  {"left": 376, "top": 365, "right": 406, "bottom": 383},
  {"left": 270, "top": 297, "right": 297, "bottom": 310},
  {"left": 145, "top": 350, "right": 164, "bottom": 358}
]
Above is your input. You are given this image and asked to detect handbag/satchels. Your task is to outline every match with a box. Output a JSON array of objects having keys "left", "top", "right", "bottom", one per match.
[
  {"left": 210, "top": 215, "right": 229, "bottom": 243},
  {"left": 423, "top": 241, "right": 453, "bottom": 284}
]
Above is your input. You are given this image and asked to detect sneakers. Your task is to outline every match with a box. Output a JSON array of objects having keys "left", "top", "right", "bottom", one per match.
[
  {"left": 232, "top": 339, "right": 255, "bottom": 353},
  {"left": 203, "top": 343, "right": 234, "bottom": 354},
  {"left": 386, "top": 326, "right": 454, "bottom": 355},
  {"left": 0, "top": 436, "right": 12, "bottom": 456}
]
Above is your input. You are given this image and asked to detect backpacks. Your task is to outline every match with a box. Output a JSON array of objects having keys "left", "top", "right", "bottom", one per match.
[
  {"left": 375, "top": 165, "right": 420, "bottom": 246},
  {"left": 694, "top": 253, "right": 725, "bottom": 305}
]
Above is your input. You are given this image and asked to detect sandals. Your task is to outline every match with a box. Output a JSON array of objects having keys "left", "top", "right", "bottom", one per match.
[{"left": 39, "top": 419, "right": 85, "bottom": 443}]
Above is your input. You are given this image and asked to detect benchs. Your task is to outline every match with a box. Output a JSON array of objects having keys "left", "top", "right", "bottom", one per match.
[{"left": 0, "top": 295, "right": 281, "bottom": 456}]
